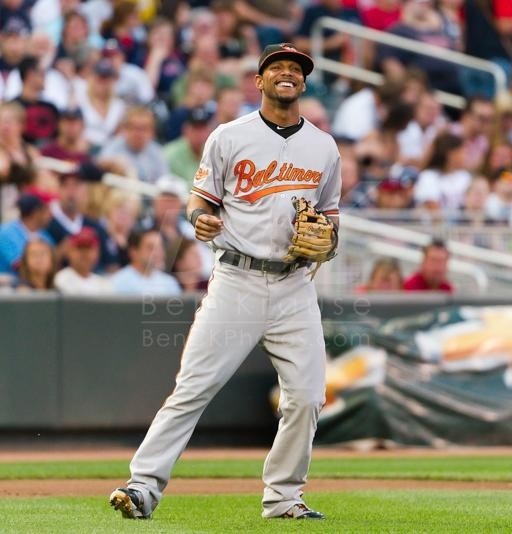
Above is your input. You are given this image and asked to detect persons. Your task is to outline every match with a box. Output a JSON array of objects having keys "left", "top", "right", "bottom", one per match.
[
  {"left": 1, "top": 0, "right": 512, "bottom": 294},
  {"left": 109, "top": 43, "right": 342, "bottom": 520}
]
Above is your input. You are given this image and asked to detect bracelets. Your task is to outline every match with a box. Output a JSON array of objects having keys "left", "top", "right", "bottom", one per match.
[{"left": 190, "top": 208, "right": 207, "bottom": 228}]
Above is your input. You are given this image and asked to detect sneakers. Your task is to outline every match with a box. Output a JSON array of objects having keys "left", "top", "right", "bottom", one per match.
[
  {"left": 280, "top": 503, "right": 324, "bottom": 518},
  {"left": 109, "top": 488, "right": 145, "bottom": 519}
]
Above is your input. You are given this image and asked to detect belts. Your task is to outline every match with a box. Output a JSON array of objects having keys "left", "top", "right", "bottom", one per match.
[{"left": 220, "top": 252, "right": 305, "bottom": 273}]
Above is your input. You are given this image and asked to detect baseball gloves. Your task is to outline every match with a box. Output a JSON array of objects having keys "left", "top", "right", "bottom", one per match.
[{"left": 278, "top": 197, "right": 338, "bottom": 282}]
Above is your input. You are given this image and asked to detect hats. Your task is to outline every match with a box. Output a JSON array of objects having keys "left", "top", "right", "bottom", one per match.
[{"left": 258, "top": 44, "right": 314, "bottom": 75}]
